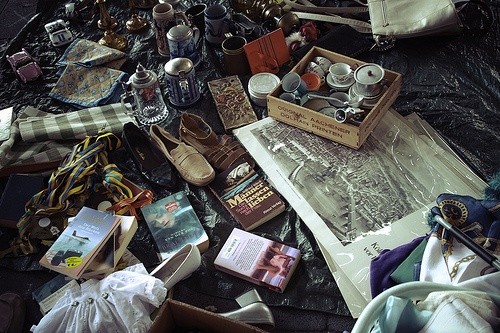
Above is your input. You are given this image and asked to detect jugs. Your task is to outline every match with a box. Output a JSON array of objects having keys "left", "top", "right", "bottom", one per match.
[
  {"left": 166, "top": 24, "right": 202, "bottom": 67},
  {"left": 163, "top": 57, "right": 201, "bottom": 109},
  {"left": 120, "top": 63, "right": 169, "bottom": 125}
]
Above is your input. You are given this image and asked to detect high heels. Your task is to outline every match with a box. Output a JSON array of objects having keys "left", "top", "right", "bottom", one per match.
[
  {"left": 215, "top": 288, "right": 276, "bottom": 331},
  {"left": 149, "top": 244, "right": 201, "bottom": 292}
]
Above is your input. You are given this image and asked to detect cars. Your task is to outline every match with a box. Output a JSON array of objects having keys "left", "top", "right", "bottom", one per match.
[
  {"left": 44, "top": 20, "right": 73, "bottom": 47},
  {"left": 6, "top": 48, "right": 42, "bottom": 83}
]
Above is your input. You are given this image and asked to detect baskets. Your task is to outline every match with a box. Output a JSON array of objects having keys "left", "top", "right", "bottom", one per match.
[{"left": 351, "top": 281, "right": 500, "bottom": 333}]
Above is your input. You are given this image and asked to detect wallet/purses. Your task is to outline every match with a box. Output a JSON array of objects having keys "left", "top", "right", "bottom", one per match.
[{"left": 243, "top": 24, "right": 373, "bottom": 79}]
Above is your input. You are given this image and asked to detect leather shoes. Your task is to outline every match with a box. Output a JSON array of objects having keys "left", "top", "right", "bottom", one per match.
[{"left": 122, "top": 122, "right": 215, "bottom": 189}]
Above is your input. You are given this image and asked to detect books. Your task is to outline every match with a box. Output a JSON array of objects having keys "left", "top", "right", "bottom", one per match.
[
  {"left": 213, "top": 227, "right": 302, "bottom": 295},
  {"left": 140, "top": 191, "right": 210, "bottom": 262},
  {"left": 205, "top": 161, "right": 287, "bottom": 230},
  {"left": 30, "top": 207, "right": 140, "bottom": 316}
]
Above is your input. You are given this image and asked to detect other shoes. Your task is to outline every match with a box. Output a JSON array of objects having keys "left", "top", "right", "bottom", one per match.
[{"left": 0, "top": 291, "right": 26, "bottom": 333}]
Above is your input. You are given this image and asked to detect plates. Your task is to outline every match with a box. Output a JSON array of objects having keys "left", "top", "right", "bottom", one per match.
[
  {"left": 326, "top": 72, "right": 354, "bottom": 90},
  {"left": 347, "top": 84, "right": 388, "bottom": 109},
  {"left": 351, "top": 83, "right": 384, "bottom": 102}
]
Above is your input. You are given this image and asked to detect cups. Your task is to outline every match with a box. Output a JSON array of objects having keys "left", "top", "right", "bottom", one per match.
[
  {"left": 184, "top": 3, "right": 207, "bottom": 35},
  {"left": 354, "top": 63, "right": 385, "bottom": 97},
  {"left": 329, "top": 62, "right": 355, "bottom": 84},
  {"left": 203, "top": 4, "right": 231, "bottom": 44},
  {"left": 282, "top": 71, "right": 307, "bottom": 98},
  {"left": 305, "top": 56, "right": 333, "bottom": 80},
  {"left": 152, "top": 3, "right": 189, "bottom": 57},
  {"left": 221, "top": 35, "right": 247, "bottom": 73},
  {"left": 300, "top": 71, "right": 325, "bottom": 91},
  {"left": 278, "top": 93, "right": 302, "bottom": 108},
  {"left": 328, "top": 92, "right": 350, "bottom": 107}
]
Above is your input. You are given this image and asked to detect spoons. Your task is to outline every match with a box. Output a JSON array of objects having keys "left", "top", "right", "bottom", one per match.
[{"left": 306, "top": 94, "right": 364, "bottom": 108}]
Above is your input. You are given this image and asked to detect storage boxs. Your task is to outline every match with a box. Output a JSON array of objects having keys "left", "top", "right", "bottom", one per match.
[
  {"left": 267, "top": 46, "right": 402, "bottom": 149},
  {"left": 147, "top": 298, "right": 270, "bottom": 333}
]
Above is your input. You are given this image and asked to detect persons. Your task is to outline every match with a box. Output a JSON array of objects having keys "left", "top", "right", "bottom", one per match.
[
  {"left": 142, "top": 205, "right": 201, "bottom": 253},
  {"left": 256, "top": 246, "right": 295, "bottom": 278}
]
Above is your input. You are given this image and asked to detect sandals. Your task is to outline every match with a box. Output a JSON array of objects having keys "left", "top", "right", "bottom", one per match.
[{"left": 179, "top": 112, "right": 255, "bottom": 173}]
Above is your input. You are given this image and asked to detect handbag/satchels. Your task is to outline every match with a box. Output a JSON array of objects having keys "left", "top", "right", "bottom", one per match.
[{"left": 282, "top": 0, "right": 463, "bottom": 51}]
